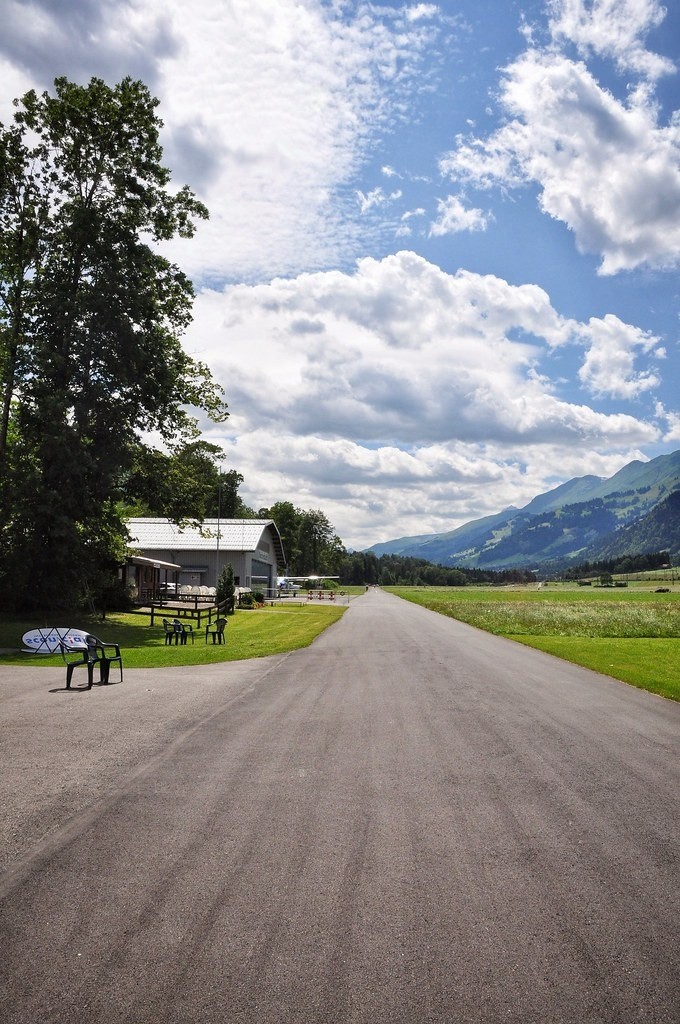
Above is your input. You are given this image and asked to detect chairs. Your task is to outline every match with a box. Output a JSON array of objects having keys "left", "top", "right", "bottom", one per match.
[
  {"left": 163, "top": 618, "right": 193, "bottom": 646},
  {"left": 205, "top": 618, "right": 228, "bottom": 644},
  {"left": 180, "top": 585, "right": 253, "bottom": 602},
  {"left": 57, "top": 635, "right": 123, "bottom": 690}
]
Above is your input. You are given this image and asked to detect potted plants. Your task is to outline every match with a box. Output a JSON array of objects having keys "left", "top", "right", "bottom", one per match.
[{"left": 255, "top": 592, "right": 264, "bottom": 607}]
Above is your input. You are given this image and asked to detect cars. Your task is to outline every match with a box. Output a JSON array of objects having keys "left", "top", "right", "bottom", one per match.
[
  {"left": 158, "top": 584, "right": 177, "bottom": 594},
  {"left": 163, "top": 582, "right": 181, "bottom": 589}
]
